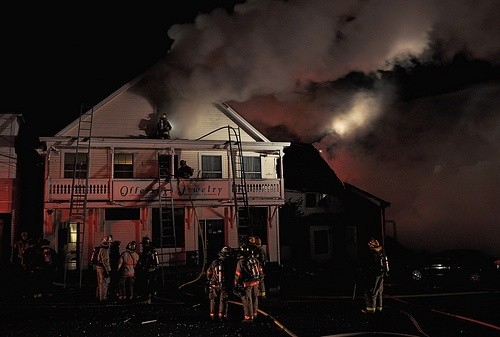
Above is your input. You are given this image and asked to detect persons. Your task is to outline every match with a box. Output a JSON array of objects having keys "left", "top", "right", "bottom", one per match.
[
  {"left": 90, "top": 235, "right": 159, "bottom": 302},
  {"left": 12, "top": 231, "right": 59, "bottom": 298},
  {"left": 156, "top": 112, "right": 171, "bottom": 139},
  {"left": 206, "top": 235, "right": 267, "bottom": 322},
  {"left": 176, "top": 160, "right": 194, "bottom": 178},
  {"left": 362, "top": 239, "right": 384, "bottom": 313}
]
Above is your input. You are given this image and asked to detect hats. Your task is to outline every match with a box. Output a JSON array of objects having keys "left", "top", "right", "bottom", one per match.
[
  {"left": 239, "top": 245, "right": 253, "bottom": 256},
  {"left": 218, "top": 247, "right": 230, "bottom": 258},
  {"left": 102, "top": 235, "right": 113, "bottom": 243},
  {"left": 142, "top": 237, "right": 150, "bottom": 247},
  {"left": 128, "top": 241, "right": 137, "bottom": 250}
]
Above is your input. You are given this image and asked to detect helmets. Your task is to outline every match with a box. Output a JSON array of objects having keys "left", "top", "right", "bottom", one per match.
[{"left": 368, "top": 239, "right": 382, "bottom": 251}]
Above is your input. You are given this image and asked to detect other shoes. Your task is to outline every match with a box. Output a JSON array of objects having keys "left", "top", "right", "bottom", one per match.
[
  {"left": 96, "top": 298, "right": 100, "bottom": 302},
  {"left": 242, "top": 318, "right": 254, "bottom": 323},
  {"left": 375, "top": 306, "right": 383, "bottom": 313},
  {"left": 210, "top": 313, "right": 215, "bottom": 320},
  {"left": 219, "top": 313, "right": 226, "bottom": 322},
  {"left": 114, "top": 299, "right": 135, "bottom": 305},
  {"left": 361, "top": 307, "right": 376, "bottom": 314},
  {"left": 99, "top": 300, "right": 108, "bottom": 305}
]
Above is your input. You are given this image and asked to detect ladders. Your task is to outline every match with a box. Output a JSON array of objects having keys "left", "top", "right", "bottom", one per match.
[
  {"left": 155, "top": 151, "right": 181, "bottom": 290},
  {"left": 61, "top": 104, "right": 93, "bottom": 289},
  {"left": 228, "top": 124, "right": 255, "bottom": 245}
]
